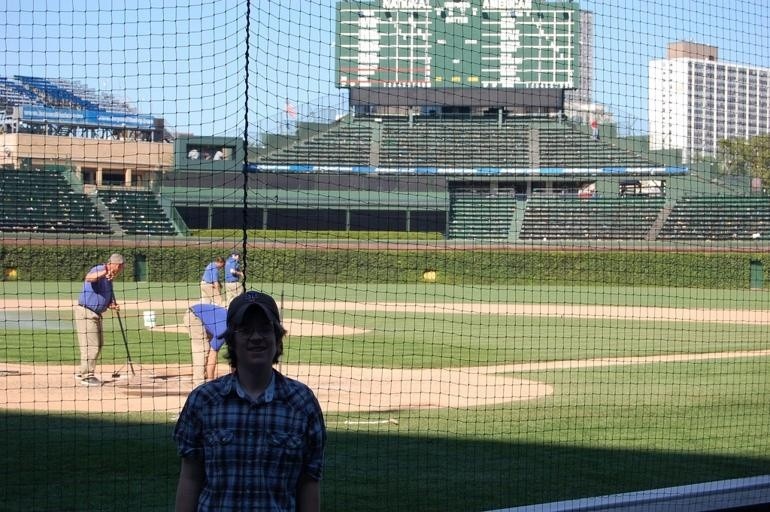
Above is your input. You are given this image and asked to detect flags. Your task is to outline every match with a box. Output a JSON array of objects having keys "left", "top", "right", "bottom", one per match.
[{"left": 285, "top": 98, "right": 297, "bottom": 119}]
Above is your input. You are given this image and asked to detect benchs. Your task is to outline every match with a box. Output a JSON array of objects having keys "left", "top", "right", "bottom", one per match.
[{"left": 1, "top": 73, "right": 190, "bottom": 240}]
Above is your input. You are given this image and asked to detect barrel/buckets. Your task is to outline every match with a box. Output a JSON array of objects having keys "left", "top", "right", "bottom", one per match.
[{"left": 142, "top": 311, "right": 155, "bottom": 328}]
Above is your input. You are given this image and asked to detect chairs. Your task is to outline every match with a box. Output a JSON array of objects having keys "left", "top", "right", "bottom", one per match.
[
  {"left": 446, "top": 188, "right": 769, "bottom": 240},
  {"left": 255, "top": 112, "right": 669, "bottom": 171}
]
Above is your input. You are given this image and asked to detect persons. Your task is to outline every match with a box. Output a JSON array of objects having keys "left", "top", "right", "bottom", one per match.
[
  {"left": 200, "top": 256, "right": 225, "bottom": 306},
  {"left": 183, "top": 303, "right": 227, "bottom": 390},
  {"left": 170, "top": 290, "right": 327, "bottom": 512},
  {"left": 76, "top": 253, "right": 126, "bottom": 387},
  {"left": 590, "top": 118, "right": 598, "bottom": 139},
  {"left": 187, "top": 144, "right": 229, "bottom": 161},
  {"left": 224, "top": 251, "right": 245, "bottom": 310}
]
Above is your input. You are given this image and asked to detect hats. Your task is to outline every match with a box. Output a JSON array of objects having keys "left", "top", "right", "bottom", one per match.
[
  {"left": 226, "top": 290, "right": 282, "bottom": 327},
  {"left": 108, "top": 253, "right": 126, "bottom": 265}
]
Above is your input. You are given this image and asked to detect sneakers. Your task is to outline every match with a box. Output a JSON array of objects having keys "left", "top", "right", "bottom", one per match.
[{"left": 75, "top": 372, "right": 104, "bottom": 387}]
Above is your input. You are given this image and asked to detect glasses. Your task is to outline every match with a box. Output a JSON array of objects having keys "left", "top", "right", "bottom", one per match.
[{"left": 233, "top": 324, "right": 276, "bottom": 340}]
